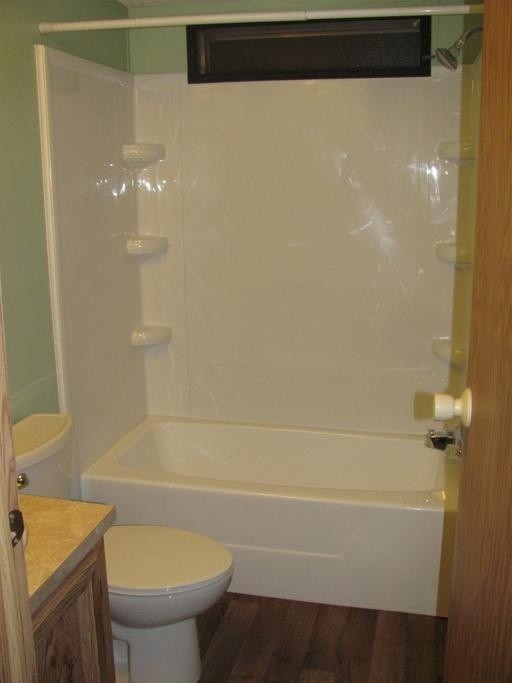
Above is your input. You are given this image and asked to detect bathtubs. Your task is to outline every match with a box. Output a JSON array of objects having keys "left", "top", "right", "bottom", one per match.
[{"left": 78, "top": 412, "right": 458, "bottom": 621}]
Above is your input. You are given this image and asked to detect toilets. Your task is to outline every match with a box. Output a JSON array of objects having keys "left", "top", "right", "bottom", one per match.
[{"left": 10, "top": 410, "right": 234, "bottom": 679}]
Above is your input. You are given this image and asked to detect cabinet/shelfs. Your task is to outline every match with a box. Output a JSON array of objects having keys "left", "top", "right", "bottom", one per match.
[{"left": 28, "top": 537, "right": 118, "bottom": 683}]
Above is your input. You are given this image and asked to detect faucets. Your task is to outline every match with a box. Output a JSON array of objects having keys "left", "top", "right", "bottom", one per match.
[{"left": 419, "top": 424, "right": 466, "bottom": 456}]
